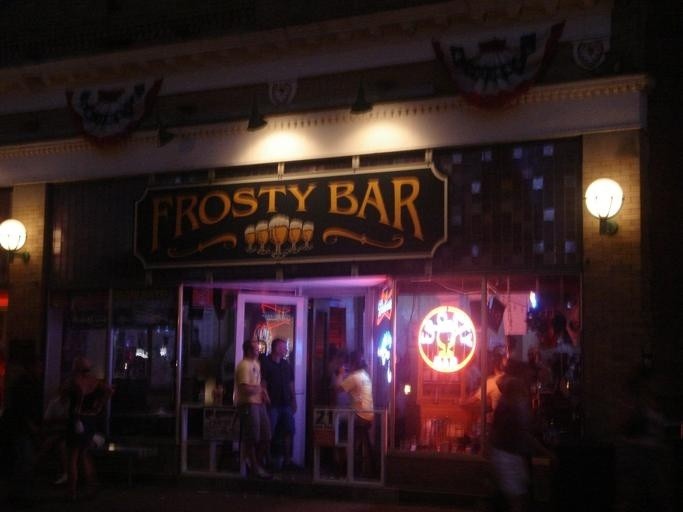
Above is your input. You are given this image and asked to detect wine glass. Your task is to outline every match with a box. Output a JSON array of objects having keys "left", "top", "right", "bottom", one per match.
[{"left": 241, "top": 216, "right": 318, "bottom": 261}]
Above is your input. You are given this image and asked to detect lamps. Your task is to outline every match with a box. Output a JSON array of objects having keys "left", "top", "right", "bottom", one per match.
[
  {"left": 584, "top": 177, "right": 624, "bottom": 235},
  {"left": 0, "top": 219, "right": 30, "bottom": 264},
  {"left": 151, "top": 70, "right": 391, "bottom": 149}
]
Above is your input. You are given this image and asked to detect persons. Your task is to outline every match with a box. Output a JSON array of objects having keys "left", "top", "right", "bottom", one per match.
[
  {"left": 0, "top": 337, "right": 419, "bottom": 512},
  {"left": 464, "top": 339, "right": 683, "bottom": 512}
]
